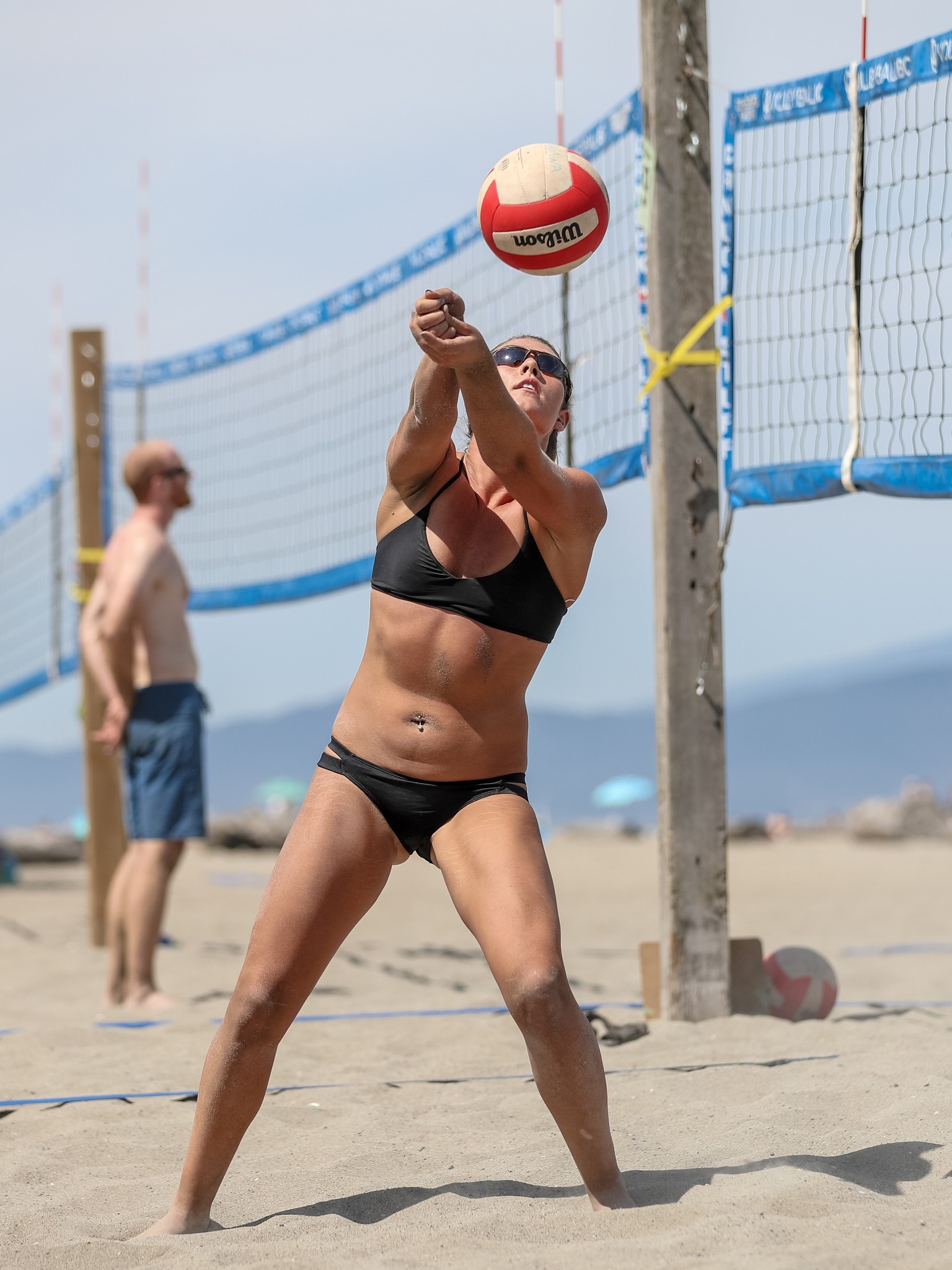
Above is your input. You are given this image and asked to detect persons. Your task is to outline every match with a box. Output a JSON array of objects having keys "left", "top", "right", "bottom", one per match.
[
  {"left": 158, "top": 292, "right": 643, "bottom": 1228},
  {"left": 78, "top": 438, "right": 209, "bottom": 1016}
]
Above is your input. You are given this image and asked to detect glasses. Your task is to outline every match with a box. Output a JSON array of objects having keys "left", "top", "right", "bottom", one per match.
[{"left": 493, "top": 345, "right": 571, "bottom": 380}]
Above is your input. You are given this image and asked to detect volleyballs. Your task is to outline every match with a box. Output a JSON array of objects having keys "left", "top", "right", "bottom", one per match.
[
  {"left": 476, "top": 143, "right": 611, "bottom": 277},
  {"left": 764, "top": 945, "right": 837, "bottom": 1023}
]
